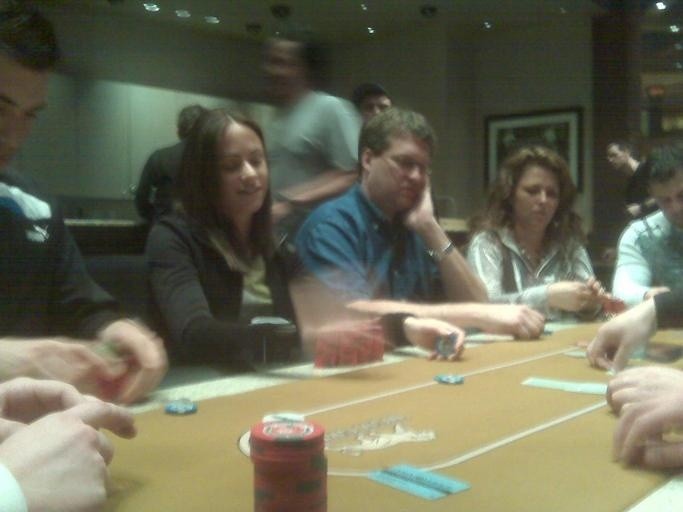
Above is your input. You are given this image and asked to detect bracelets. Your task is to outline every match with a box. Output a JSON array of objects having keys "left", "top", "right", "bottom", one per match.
[
  {"left": 426, "top": 239, "right": 454, "bottom": 266},
  {"left": 274, "top": 190, "right": 297, "bottom": 207}
]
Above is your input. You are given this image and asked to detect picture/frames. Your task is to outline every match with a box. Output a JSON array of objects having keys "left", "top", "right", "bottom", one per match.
[{"left": 483, "top": 103, "right": 584, "bottom": 193}]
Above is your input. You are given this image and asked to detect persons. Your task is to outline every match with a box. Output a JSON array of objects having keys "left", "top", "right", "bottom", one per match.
[
  {"left": 130, "top": 104, "right": 214, "bottom": 248},
  {"left": 348, "top": 80, "right": 395, "bottom": 126},
  {"left": 608, "top": 389, "right": 682, "bottom": 473},
  {"left": 469, "top": 144, "right": 614, "bottom": 322},
  {"left": 602, "top": 134, "right": 659, "bottom": 218},
  {"left": 0, "top": 4, "right": 170, "bottom": 409},
  {"left": 0, "top": 376, "right": 137, "bottom": 512},
  {"left": 140, "top": 106, "right": 468, "bottom": 369},
  {"left": 601, "top": 144, "right": 682, "bottom": 325},
  {"left": 585, "top": 288, "right": 683, "bottom": 422},
  {"left": 295, "top": 106, "right": 545, "bottom": 340},
  {"left": 237, "top": 31, "right": 371, "bottom": 278}
]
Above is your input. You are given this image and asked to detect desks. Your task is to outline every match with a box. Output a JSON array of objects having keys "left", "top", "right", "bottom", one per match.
[{"left": 97, "top": 311, "right": 683, "bottom": 512}]
